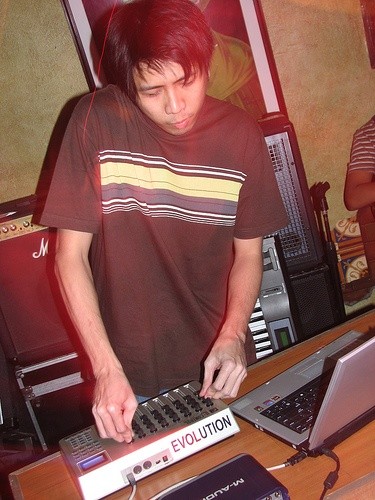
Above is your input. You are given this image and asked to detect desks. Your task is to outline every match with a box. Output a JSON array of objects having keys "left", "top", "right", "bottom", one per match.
[{"left": 7, "top": 307, "right": 375, "bottom": 499}]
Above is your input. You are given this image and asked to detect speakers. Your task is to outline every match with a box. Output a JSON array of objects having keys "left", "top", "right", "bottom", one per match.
[{"left": 260, "top": 121, "right": 345, "bottom": 338}]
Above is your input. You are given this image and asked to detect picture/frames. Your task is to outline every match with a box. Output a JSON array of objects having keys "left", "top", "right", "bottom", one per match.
[{"left": 58, "top": 1, "right": 292, "bottom": 134}]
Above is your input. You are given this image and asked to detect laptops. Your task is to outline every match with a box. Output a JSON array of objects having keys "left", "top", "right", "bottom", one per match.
[{"left": 229, "top": 327, "right": 375, "bottom": 457}]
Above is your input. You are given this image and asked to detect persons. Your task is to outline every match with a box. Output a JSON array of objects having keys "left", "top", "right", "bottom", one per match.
[
  {"left": 41, "top": 1, "right": 291, "bottom": 444},
  {"left": 342, "top": 112, "right": 375, "bottom": 211}
]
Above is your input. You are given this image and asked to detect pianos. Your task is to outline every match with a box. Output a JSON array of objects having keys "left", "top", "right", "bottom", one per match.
[{"left": 245, "top": 297, "right": 273, "bottom": 361}]
